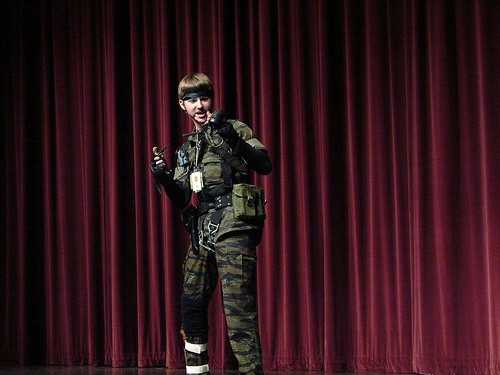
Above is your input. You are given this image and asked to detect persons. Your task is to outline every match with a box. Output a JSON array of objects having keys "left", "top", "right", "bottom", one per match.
[{"left": 148, "top": 71, "right": 272, "bottom": 374}]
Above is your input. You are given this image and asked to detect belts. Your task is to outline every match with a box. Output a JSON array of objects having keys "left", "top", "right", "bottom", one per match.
[{"left": 188, "top": 194, "right": 233, "bottom": 221}]
